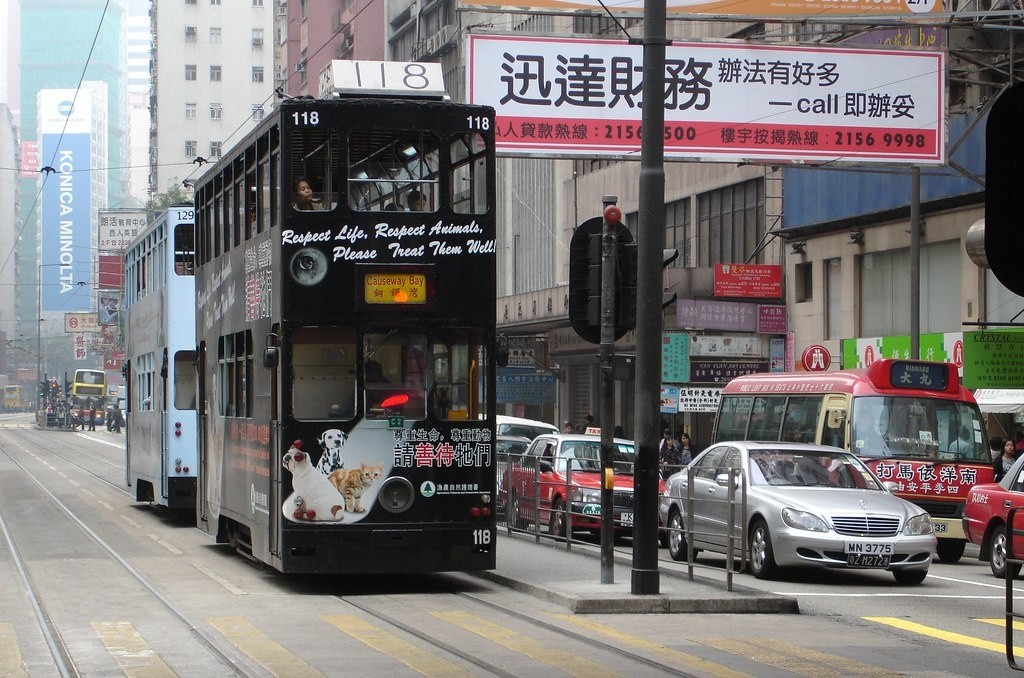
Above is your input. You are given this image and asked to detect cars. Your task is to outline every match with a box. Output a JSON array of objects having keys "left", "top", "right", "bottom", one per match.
[
  {"left": 963, "top": 449, "right": 1024, "bottom": 578},
  {"left": 658, "top": 440, "right": 937, "bottom": 584},
  {"left": 109, "top": 398, "right": 125, "bottom": 423},
  {"left": 501, "top": 426, "right": 665, "bottom": 542}
]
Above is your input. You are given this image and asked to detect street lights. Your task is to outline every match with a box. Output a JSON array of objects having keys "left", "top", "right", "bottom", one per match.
[{"left": 36, "top": 263, "right": 70, "bottom": 423}]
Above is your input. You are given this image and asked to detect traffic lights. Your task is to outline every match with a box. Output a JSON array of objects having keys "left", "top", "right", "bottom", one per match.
[
  {"left": 964, "top": 81, "right": 1023, "bottom": 301},
  {"left": 624, "top": 244, "right": 680, "bottom": 330},
  {"left": 66, "top": 381, "right": 72, "bottom": 392},
  {"left": 40, "top": 381, "right": 50, "bottom": 398},
  {"left": 51, "top": 382, "right": 62, "bottom": 398}
]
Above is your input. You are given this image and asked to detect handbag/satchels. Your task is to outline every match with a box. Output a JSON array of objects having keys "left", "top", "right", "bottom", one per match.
[{"left": 120, "top": 418, "right": 125, "bottom": 427}]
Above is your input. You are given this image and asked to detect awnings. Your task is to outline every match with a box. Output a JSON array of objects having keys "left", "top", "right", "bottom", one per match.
[{"left": 973, "top": 389, "right": 1024, "bottom": 413}]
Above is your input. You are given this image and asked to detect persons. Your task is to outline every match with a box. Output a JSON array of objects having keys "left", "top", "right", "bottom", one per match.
[
  {"left": 52, "top": 400, "right": 84, "bottom": 431},
  {"left": 659, "top": 428, "right": 695, "bottom": 480},
  {"left": 143, "top": 395, "right": 151, "bottom": 405},
  {"left": 106, "top": 404, "right": 124, "bottom": 433},
  {"left": 385, "top": 202, "right": 404, "bottom": 211},
  {"left": 292, "top": 177, "right": 324, "bottom": 211},
  {"left": 949, "top": 425, "right": 973, "bottom": 459},
  {"left": 578, "top": 415, "right": 596, "bottom": 434},
  {"left": 7, "top": 399, "right": 37, "bottom": 414},
  {"left": 614, "top": 425, "right": 626, "bottom": 439},
  {"left": 87, "top": 402, "right": 96, "bottom": 431},
  {"left": 333, "top": 167, "right": 369, "bottom": 211},
  {"left": 328, "top": 361, "right": 435, "bottom": 419},
  {"left": 408, "top": 191, "right": 426, "bottom": 211},
  {"left": 613, "top": 455, "right": 630, "bottom": 473},
  {"left": 754, "top": 458, "right": 773, "bottom": 483},
  {"left": 981, "top": 436, "right": 1019, "bottom": 484},
  {"left": 562, "top": 422, "right": 573, "bottom": 434}
]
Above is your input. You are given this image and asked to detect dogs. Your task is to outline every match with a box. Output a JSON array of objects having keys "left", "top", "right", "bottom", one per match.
[
  {"left": 315, "top": 429, "right": 348, "bottom": 478},
  {"left": 282, "top": 444, "right": 344, "bottom": 521}
]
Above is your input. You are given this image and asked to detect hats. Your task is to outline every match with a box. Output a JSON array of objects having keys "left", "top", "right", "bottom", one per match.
[{"left": 107, "top": 406, "right": 112, "bottom": 409}]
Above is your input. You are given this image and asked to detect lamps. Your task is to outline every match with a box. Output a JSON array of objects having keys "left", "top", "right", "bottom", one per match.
[
  {"left": 790, "top": 242, "right": 806, "bottom": 254},
  {"left": 905, "top": 220, "right": 926, "bottom": 236},
  {"left": 847, "top": 233, "right": 865, "bottom": 245}
]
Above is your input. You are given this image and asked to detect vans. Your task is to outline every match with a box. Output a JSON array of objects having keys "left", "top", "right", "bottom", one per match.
[{"left": 477, "top": 411, "right": 559, "bottom": 512}]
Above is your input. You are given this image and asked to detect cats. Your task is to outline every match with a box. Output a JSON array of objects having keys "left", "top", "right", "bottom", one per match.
[{"left": 326, "top": 458, "right": 384, "bottom": 512}]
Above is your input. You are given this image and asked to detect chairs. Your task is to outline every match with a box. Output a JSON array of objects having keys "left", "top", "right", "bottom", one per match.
[{"left": 735, "top": 419, "right": 800, "bottom": 441}]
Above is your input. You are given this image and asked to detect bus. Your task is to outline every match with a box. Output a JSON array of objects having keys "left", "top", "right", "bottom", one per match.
[
  {"left": 4, "top": 384, "right": 23, "bottom": 413},
  {"left": 73, "top": 369, "right": 108, "bottom": 425},
  {"left": 710, "top": 359, "right": 993, "bottom": 563},
  {"left": 122, "top": 205, "right": 196, "bottom": 508},
  {"left": 191, "top": 60, "right": 497, "bottom": 575}
]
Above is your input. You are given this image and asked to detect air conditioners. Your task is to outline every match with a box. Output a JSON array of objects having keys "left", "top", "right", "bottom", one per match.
[
  {"left": 252, "top": 37, "right": 262, "bottom": 44},
  {"left": 186, "top": 26, "right": 196, "bottom": 34},
  {"left": 429, "top": 25, "right": 458, "bottom": 54}
]
